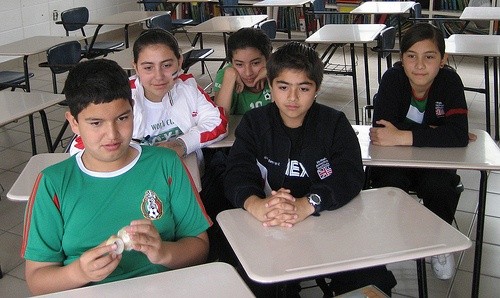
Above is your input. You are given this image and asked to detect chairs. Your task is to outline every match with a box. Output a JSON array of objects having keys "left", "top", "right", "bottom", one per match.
[{"left": 0, "top": 0, "right": 500, "bottom": 297}]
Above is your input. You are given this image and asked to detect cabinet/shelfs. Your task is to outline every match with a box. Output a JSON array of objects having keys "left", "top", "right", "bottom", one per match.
[
  {"left": 141, "top": 0, "right": 497, "bottom": 56},
  {"left": 187, "top": 15, "right": 268, "bottom": 74}
]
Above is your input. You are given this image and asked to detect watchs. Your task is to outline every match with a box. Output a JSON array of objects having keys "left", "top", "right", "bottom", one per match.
[{"left": 304, "top": 192, "right": 321, "bottom": 216}]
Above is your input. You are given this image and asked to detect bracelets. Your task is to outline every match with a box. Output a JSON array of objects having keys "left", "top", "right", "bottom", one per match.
[{"left": 178, "top": 137, "right": 187, "bottom": 159}]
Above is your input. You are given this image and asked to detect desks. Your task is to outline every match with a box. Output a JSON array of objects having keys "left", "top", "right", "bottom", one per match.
[
  {"left": 444, "top": 33, "right": 500, "bottom": 142},
  {"left": 0, "top": 90, "right": 67, "bottom": 156},
  {"left": 349, "top": 2, "right": 415, "bottom": 49},
  {"left": 85, "top": 11, "right": 171, "bottom": 76},
  {"left": 253, "top": 0, "right": 312, "bottom": 47},
  {"left": 204, "top": 113, "right": 244, "bottom": 148},
  {"left": 0, "top": 35, "right": 85, "bottom": 95},
  {"left": 349, "top": 125, "right": 500, "bottom": 298},
  {"left": 216, "top": 187, "right": 473, "bottom": 298},
  {"left": 29, "top": 262, "right": 258, "bottom": 298},
  {"left": 305, "top": 24, "right": 386, "bottom": 124},
  {"left": 459, "top": 6, "right": 500, "bottom": 34},
  {"left": 6, "top": 152, "right": 201, "bottom": 201},
  {"left": 105, "top": 48, "right": 136, "bottom": 70}
]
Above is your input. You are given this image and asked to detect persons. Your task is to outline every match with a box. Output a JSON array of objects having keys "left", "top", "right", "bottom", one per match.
[
  {"left": 364, "top": 23, "right": 476, "bottom": 278},
  {"left": 21, "top": 29, "right": 398, "bottom": 298}
]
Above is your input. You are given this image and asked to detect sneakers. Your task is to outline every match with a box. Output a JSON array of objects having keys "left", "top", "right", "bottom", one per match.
[{"left": 431, "top": 253, "right": 455, "bottom": 280}]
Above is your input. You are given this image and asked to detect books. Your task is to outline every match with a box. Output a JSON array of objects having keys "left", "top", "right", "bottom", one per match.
[{"left": 161, "top": 0, "right": 489, "bottom": 37}]
